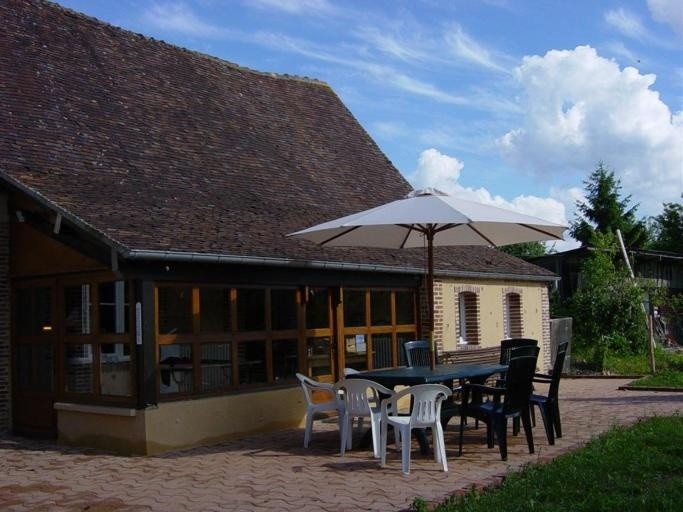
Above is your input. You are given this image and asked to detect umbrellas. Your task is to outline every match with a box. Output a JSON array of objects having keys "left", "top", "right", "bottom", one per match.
[{"left": 285, "top": 187, "right": 570, "bottom": 369}]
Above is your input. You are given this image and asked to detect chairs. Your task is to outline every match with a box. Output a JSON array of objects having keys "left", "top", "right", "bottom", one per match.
[{"left": 295, "top": 340, "right": 569, "bottom": 475}]
